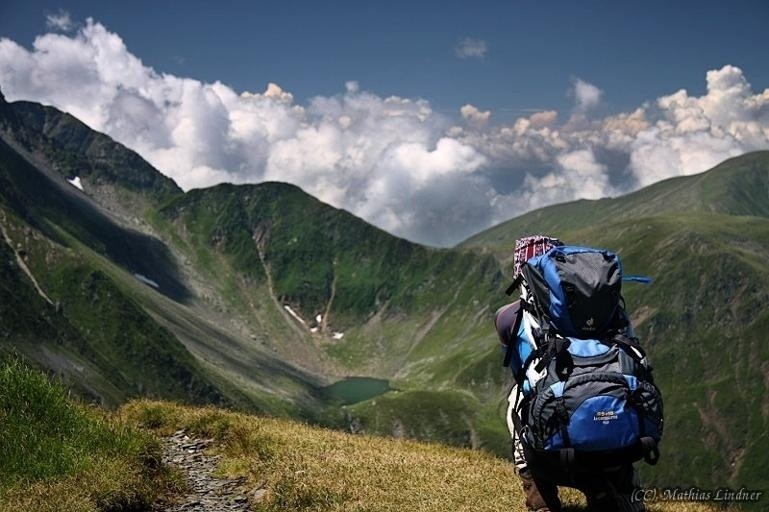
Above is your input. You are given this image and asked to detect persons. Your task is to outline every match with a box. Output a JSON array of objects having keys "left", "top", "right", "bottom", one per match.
[{"left": 493, "top": 233, "right": 647, "bottom": 512}]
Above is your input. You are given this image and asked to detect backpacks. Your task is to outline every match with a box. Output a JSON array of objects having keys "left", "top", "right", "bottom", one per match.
[{"left": 518, "top": 246, "right": 664, "bottom": 469}]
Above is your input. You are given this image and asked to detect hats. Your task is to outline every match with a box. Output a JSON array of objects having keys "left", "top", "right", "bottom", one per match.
[{"left": 513, "top": 235, "right": 564, "bottom": 279}]
